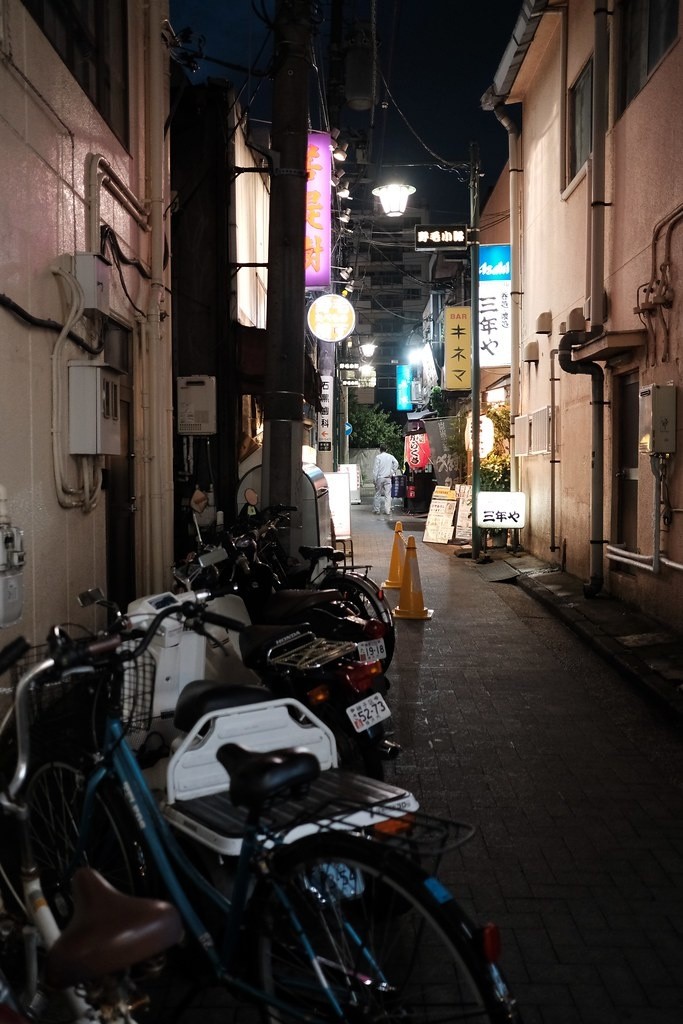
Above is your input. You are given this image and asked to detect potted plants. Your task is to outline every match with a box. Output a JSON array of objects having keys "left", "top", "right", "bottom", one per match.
[{"left": 475, "top": 448, "right": 513, "bottom": 550}]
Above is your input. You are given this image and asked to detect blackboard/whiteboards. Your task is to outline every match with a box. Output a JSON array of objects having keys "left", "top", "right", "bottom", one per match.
[
  {"left": 321, "top": 472, "right": 351, "bottom": 538},
  {"left": 337, "top": 463, "right": 361, "bottom": 504}
]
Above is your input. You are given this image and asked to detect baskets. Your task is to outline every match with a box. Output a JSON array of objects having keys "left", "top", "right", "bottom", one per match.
[{"left": 11, "top": 635, "right": 158, "bottom": 733}]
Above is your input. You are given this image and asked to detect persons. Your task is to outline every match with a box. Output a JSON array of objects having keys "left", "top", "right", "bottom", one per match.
[{"left": 372, "top": 444, "right": 399, "bottom": 515}]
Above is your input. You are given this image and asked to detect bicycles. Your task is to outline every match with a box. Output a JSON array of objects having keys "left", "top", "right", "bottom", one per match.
[
  {"left": 0, "top": 601, "right": 518, "bottom": 1024},
  {"left": 52, "top": 486, "right": 406, "bottom": 1021},
  {"left": 0, "top": 632, "right": 189, "bottom": 1024}
]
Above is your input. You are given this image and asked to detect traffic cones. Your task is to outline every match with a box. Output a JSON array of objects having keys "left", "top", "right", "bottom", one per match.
[
  {"left": 390, "top": 535, "right": 435, "bottom": 621},
  {"left": 380, "top": 520, "right": 408, "bottom": 589}
]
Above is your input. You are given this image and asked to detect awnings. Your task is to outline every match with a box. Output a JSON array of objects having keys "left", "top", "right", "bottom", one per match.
[{"left": 236, "top": 325, "right": 322, "bottom": 412}]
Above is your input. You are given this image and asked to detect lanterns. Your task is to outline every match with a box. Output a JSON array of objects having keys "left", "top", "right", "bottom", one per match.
[{"left": 405, "top": 434, "right": 431, "bottom": 469}]
